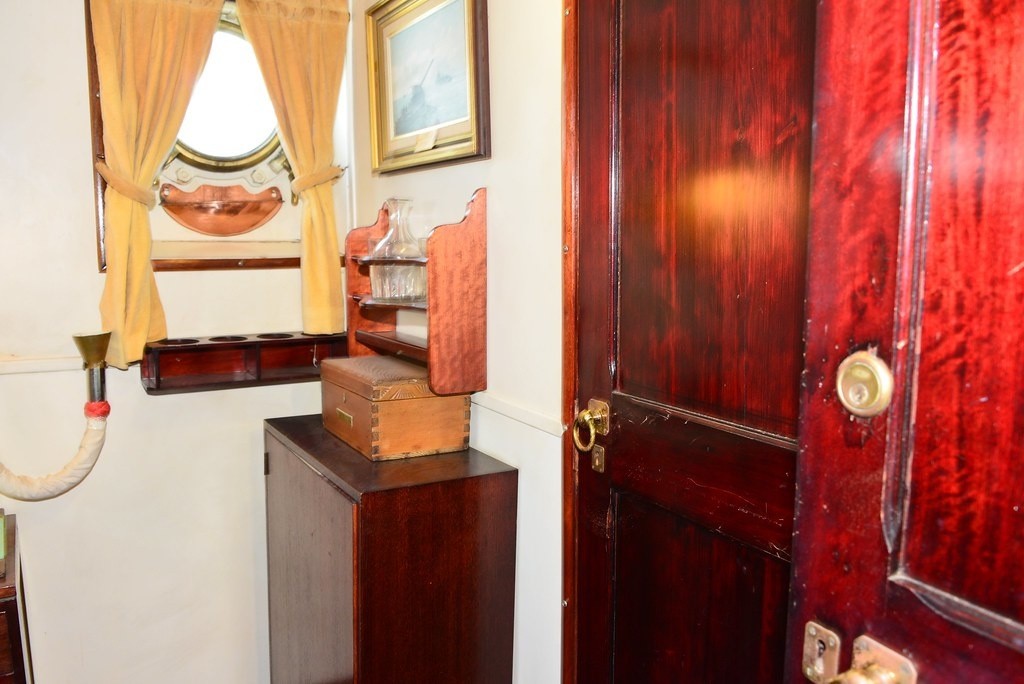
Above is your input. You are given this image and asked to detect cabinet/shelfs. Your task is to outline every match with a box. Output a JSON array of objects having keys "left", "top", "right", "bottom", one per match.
[
  {"left": 345, "top": 187, "right": 488, "bottom": 397},
  {"left": 264, "top": 413, "right": 518, "bottom": 684}
]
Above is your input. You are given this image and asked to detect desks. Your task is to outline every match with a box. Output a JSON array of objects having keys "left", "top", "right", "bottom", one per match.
[{"left": 0, "top": 513, "right": 35, "bottom": 684}]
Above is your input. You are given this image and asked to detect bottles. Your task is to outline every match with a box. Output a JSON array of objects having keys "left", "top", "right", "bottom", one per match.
[{"left": 369, "top": 198, "right": 422, "bottom": 301}]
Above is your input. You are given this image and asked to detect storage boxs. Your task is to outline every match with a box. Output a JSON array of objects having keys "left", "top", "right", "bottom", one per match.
[{"left": 321, "top": 355, "right": 471, "bottom": 462}]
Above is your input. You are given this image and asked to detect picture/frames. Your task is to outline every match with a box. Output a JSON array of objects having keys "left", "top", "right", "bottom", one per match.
[
  {"left": 84, "top": 0, "right": 303, "bottom": 273},
  {"left": 365, "top": 0, "right": 491, "bottom": 175}
]
